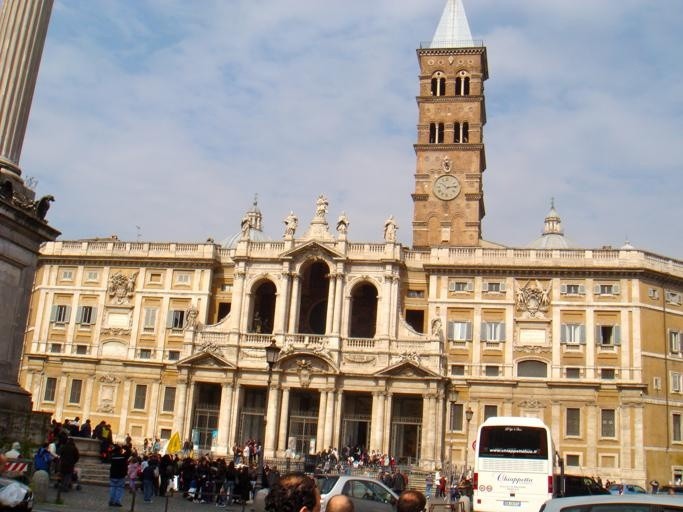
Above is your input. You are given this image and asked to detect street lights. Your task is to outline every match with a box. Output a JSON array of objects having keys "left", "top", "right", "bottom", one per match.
[
  {"left": 250, "top": 338, "right": 283, "bottom": 502},
  {"left": 445, "top": 384, "right": 461, "bottom": 508},
  {"left": 464, "top": 405, "right": 474, "bottom": 477}
]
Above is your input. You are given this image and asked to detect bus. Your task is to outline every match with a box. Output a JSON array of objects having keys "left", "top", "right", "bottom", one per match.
[{"left": 470, "top": 414, "right": 566, "bottom": 511}]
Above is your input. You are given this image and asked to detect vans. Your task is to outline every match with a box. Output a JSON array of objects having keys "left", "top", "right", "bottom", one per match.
[{"left": 554, "top": 474, "right": 611, "bottom": 499}]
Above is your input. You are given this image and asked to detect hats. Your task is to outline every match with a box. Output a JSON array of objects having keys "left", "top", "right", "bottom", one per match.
[{"left": 12, "top": 442, "right": 20, "bottom": 449}]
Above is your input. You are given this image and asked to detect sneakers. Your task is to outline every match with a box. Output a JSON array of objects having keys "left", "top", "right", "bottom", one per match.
[
  {"left": 109, "top": 502, "right": 121, "bottom": 506},
  {"left": 193, "top": 499, "right": 206, "bottom": 504},
  {"left": 216, "top": 502, "right": 226, "bottom": 507}
]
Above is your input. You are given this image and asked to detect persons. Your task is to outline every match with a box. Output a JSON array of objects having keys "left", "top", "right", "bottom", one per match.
[
  {"left": 590, "top": 472, "right": 682, "bottom": 498},
  {"left": 253, "top": 309, "right": 263, "bottom": 334},
  {"left": 263, "top": 316, "right": 272, "bottom": 334}
]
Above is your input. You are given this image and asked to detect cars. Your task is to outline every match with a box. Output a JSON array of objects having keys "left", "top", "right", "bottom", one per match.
[
  {"left": 307, "top": 472, "right": 400, "bottom": 512},
  {"left": 538, "top": 492, "right": 682, "bottom": 512},
  {"left": 608, "top": 483, "right": 649, "bottom": 494}
]
